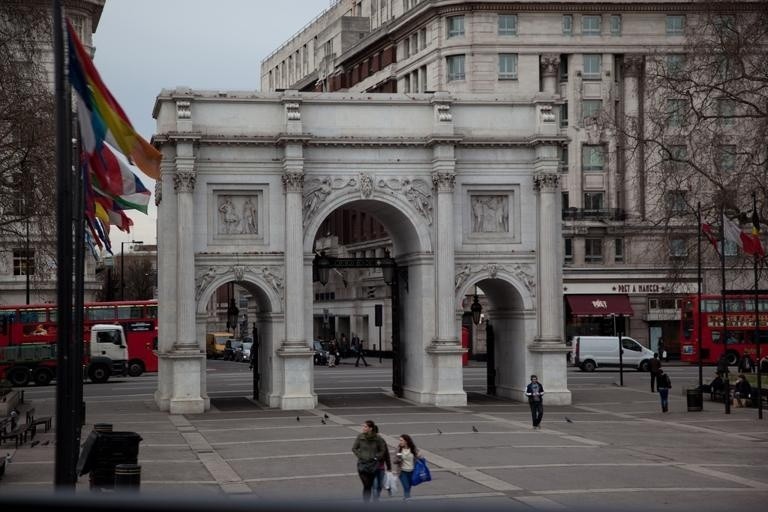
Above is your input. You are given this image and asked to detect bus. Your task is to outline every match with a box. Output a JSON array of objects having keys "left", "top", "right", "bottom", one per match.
[
  {"left": 0, "top": 299, "right": 159, "bottom": 383},
  {"left": 679, "top": 290, "right": 768, "bottom": 366}
]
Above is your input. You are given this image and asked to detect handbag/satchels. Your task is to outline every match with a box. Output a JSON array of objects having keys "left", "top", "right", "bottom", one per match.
[
  {"left": 411, "top": 456, "right": 431, "bottom": 487},
  {"left": 382, "top": 471, "right": 391, "bottom": 491},
  {"left": 388, "top": 471, "right": 399, "bottom": 496}
]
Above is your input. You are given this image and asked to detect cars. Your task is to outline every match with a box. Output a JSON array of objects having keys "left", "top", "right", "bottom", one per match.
[
  {"left": 223, "top": 337, "right": 255, "bottom": 361},
  {"left": 313, "top": 341, "right": 340, "bottom": 365}
]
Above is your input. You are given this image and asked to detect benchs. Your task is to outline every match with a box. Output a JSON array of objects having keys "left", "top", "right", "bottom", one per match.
[
  {"left": 0, "top": 407, "right": 52, "bottom": 476},
  {"left": 710, "top": 383, "right": 768, "bottom": 408}
]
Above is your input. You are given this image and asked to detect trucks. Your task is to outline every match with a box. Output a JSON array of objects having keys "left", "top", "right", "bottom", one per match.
[{"left": 0, "top": 323, "right": 130, "bottom": 388}]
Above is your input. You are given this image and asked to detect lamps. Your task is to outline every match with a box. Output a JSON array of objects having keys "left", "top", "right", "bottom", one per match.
[
  {"left": 226, "top": 283, "right": 240, "bottom": 331},
  {"left": 470, "top": 283, "right": 482, "bottom": 326},
  {"left": 315, "top": 222, "right": 331, "bottom": 285},
  {"left": 383, "top": 248, "right": 408, "bottom": 286}
]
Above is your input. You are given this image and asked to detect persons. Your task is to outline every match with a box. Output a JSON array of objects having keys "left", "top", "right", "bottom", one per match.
[
  {"left": 472, "top": 195, "right": 485, "bottom": 232},
  {"left": 656, "top": 370, "right": 673, "bottom": 414},
  {"left": 718, "top": 299, "right": 747, "bottom": 313},
  {"left": 493, "top": 195, "right": 507, "bottom": 233},
  {"left": 646, "top": 352, "right": 662, "bottom": 393},
  {"left": 31, "top": 323, "right": 48, "bottom": 337},
  {"left": 313, "top": 332, "right": 372, "bottom": 369},
  {"left": 218, "top": 195, "right": 240, "bottom": 235},
  {"left": 371, "top": 425, "right": 392, "bottom": 502},
  {"left": 524, "top": 375, "right": 545, "bottom": 430},
  {"left": 351, "top": 420, "right": 387, "bottom": 504},
  {"left": 706, "top": 331, "right": 768, "bottom": 408},
  {"left": 392, "top": 434, "right": 422, "bottom": 504},
  {"left": 243, "top": 195, "right": 258, "bottom": 235}
]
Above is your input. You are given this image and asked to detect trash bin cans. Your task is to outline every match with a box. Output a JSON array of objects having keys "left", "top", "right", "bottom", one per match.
[
  {"left": 687, "top": 388, "right": 703, "bottom": 412},
  {"left": 76, "top": 431, "right": 143, "bottom": 491}
]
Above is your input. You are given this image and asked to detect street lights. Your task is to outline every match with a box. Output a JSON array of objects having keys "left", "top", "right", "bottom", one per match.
[{"left": 120, "top": 241, "right": 144, "bottom": 300}]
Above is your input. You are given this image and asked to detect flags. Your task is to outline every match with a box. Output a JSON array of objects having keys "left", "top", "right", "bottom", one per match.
[
  {"left": 722, "top": 212, "right": 757, "bottom": 257},
  {"left": 700, "top": 208, "right": 720, "bottom": 257},
  {"left": 62, "top": 14, "right": 166, "bottom": 260},
  {"left": 751, "top": 200, "right": 765, "bottom": 258}
]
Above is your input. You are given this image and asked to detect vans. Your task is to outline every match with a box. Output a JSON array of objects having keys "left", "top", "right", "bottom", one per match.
[
  {"left": 571, "top": 335, "right": 661, "bottom": 373},
  {"left": 205, "top": 331, "right": 234, "bottom": 361}
]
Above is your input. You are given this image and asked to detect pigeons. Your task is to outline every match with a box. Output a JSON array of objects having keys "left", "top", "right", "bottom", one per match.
[
  {"left": 325, "top": 414, "right": 328, "bottom": 418},
  {"left": 565, "top": 417, "right": 573, "bottom": 423},
  {"left": 41, "top": 440, "right": 50, "bottom": 446},
  {"left": 472, "top": 426, "right": 478, "bottom": 432},
  {"left": 30, "top": 440, "right": 40, "bottom": 449},
  {"left": 297, "top": 416, "right": 299, "bottom": 421},
  {"left": 321, "top": 420, "right": 327, "bottom": 423},
  {"left": 436, "top": 427, "right": 442, "bottom": 435}
]
